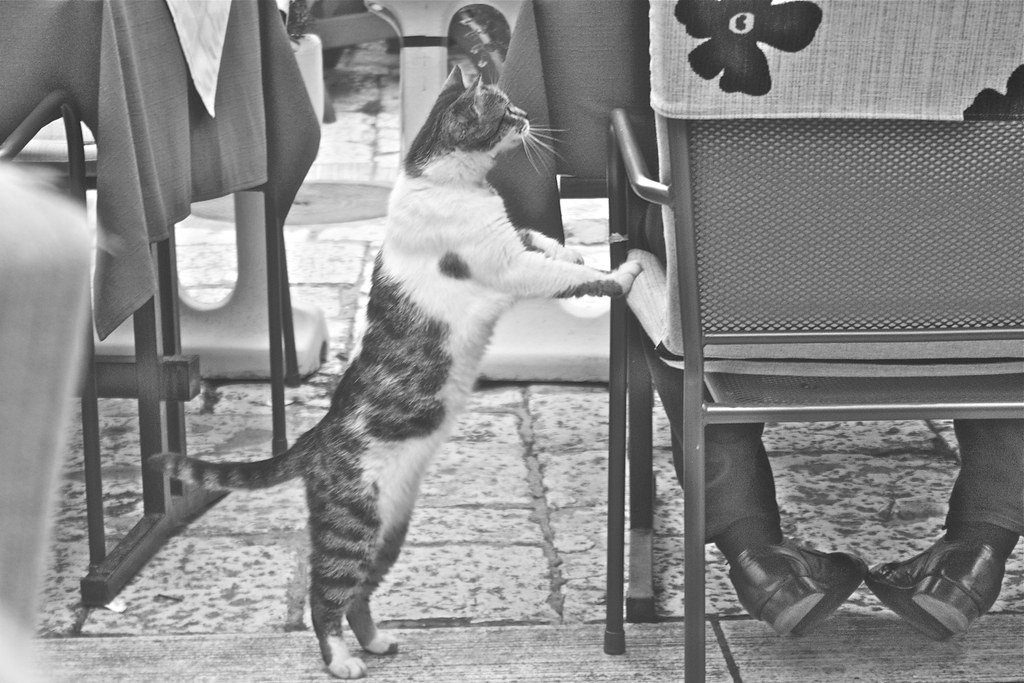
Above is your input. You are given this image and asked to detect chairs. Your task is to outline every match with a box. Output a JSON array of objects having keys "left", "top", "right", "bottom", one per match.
[{"left": 602, "top": 0, "right": 1023, "bottom": 683}]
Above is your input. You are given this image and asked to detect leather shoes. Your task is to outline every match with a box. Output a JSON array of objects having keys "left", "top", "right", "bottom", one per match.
[
  {"left": 729, "top": 537, "right": 869, "bottom": 637},
  {"left": 865, "top": 534, "right": 1005, "bottom": 641}
]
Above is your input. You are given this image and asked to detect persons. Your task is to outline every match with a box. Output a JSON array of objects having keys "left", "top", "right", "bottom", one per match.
[{"left": 629, "top": 174, "right": 1023, "bottom": 643}]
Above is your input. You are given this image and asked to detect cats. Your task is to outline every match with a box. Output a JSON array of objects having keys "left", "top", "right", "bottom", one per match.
[{"left": 144, "top": 60, "right": 641, "bottom": 679}]
